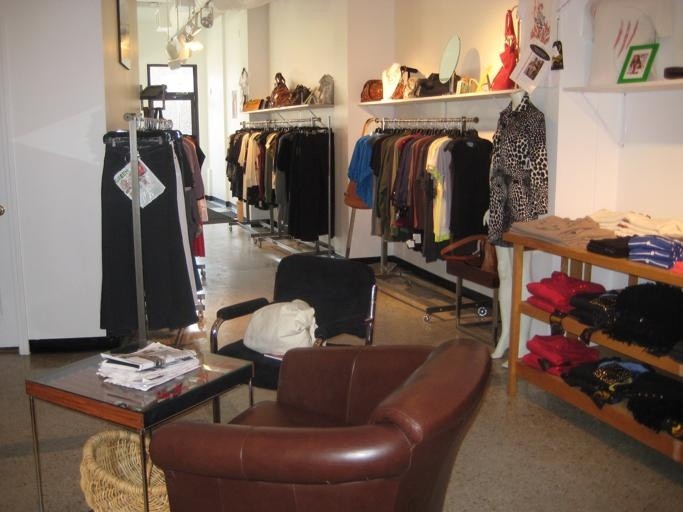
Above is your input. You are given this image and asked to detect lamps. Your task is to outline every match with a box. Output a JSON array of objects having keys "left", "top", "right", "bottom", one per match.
[{"left": 165, "top": 1, "right": 218, "bottom": 70}]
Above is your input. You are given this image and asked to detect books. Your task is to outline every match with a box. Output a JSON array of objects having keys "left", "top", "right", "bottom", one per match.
[{"left": 95, "top": 341, "right": 200, "bottom": 392}]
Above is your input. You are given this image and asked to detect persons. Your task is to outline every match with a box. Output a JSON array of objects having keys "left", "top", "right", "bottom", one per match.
[{"left": 629, "top": 54, "right": 642, "bottom": 74}]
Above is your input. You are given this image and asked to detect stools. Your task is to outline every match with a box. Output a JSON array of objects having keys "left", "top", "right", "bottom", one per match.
[{"left": 446, "top": 259, "right": 500, "bottom": 348}]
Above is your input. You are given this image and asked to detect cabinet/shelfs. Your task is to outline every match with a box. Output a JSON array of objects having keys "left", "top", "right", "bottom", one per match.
[{"left": 502, "top": 220, "right": 683, "bottom": 466}]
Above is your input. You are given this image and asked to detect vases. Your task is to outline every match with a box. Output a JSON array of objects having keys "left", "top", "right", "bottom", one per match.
[{"left": 616, "top": 44, "right": 660, "bottom": 84}]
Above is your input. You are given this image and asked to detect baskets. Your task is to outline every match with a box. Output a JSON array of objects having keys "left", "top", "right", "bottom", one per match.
[{"left": 79, "top": 428, "right": 169, "bottom": 512}]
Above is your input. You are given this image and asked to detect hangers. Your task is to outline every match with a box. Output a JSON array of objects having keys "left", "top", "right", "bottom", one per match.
[
  {"left": 241, "top": 120, "right": 328, "bottom": 134},
  {"left": 369, "top": 117, "right": 481, "bottom": 152},
  {"left": 102, "top": 113, "right": 174, "bottom": 145}
]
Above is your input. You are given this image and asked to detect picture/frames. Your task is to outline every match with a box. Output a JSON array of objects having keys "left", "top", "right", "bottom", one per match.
[{"left": 117, "top": 0, "right": 131, "bottom": 70}]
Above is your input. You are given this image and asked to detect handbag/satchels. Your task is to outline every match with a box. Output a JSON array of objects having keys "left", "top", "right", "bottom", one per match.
[
  {"left": 489, "top": 39, "right": 518, "bottom": 91},
  {"left": 242, "top": 299, "right": 318, "bottom": 357},
  {"left": 361, "top": 63, "right": 449, "bottom": 102},
  {"left": 446, "top": 235, "right": 499, "bottom": 289},
  {"left": 243, "top": 78, "right": 335, "bottom": 112}
]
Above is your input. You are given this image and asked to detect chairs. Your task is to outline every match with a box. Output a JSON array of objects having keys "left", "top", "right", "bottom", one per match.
[
  {"left": 149, "top": 338, "right": 491, "bottom": 511},
  {"left": 210, "top": 253, "right": 376, "bottom": 424}
]
